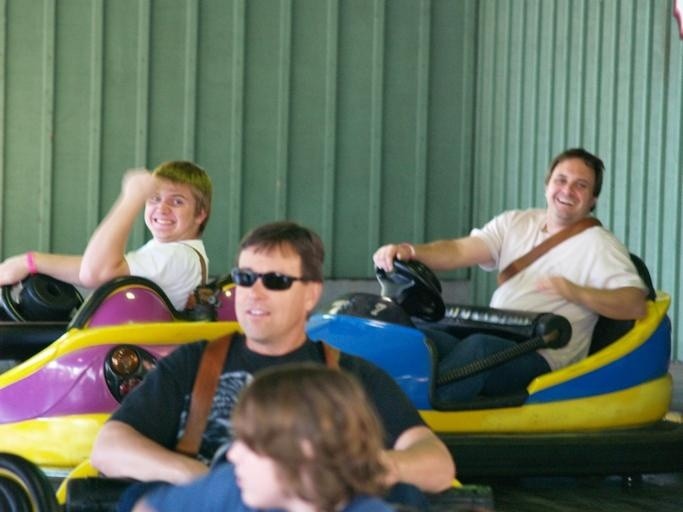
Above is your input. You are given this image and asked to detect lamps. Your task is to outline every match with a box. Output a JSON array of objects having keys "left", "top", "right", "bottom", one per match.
[{"left": 601, "top": 254, "right": 656, "bottom": 330}]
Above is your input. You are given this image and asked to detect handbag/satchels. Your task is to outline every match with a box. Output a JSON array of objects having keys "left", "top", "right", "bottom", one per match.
[
  {"left": 26, "top": 252, "right": 36, "bottom": 275},
  {"left": 400, "top": 241, "right": 417, "bottom": 262}
]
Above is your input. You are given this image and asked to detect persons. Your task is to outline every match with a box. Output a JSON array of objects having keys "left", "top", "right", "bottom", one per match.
[
  {"left": 1, "top": 161, "right": 212, "bottom": 312},
  {"left": 372, "top": 150, "right": 650, "bottom": 403},
  {"left": 138, "top": 363, "right": 400, "bottom": 512},
  {"left": 91, "top": 224, "right": 457, "bottom": 512}
]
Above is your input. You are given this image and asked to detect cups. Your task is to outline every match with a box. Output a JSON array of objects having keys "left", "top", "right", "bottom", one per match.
[{"left": 231, "top": 267, "right": 305, "bottom": 291}]
